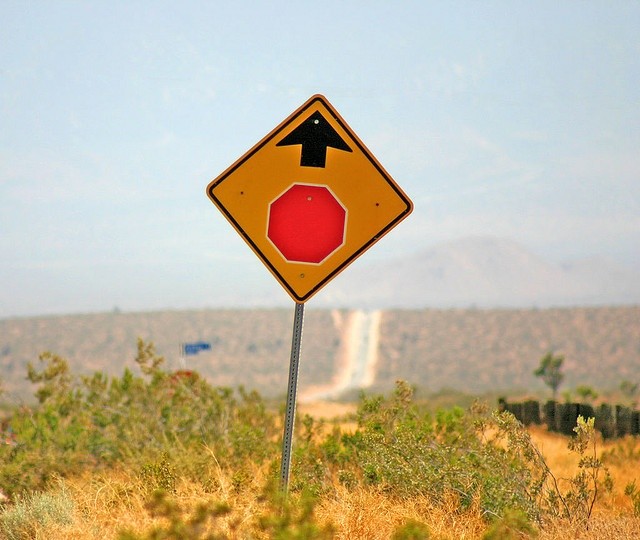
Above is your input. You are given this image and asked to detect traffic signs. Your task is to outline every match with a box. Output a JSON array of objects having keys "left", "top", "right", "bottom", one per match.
[{"left": 206, "top": 94, "right": 414, "bottom": 305}]
